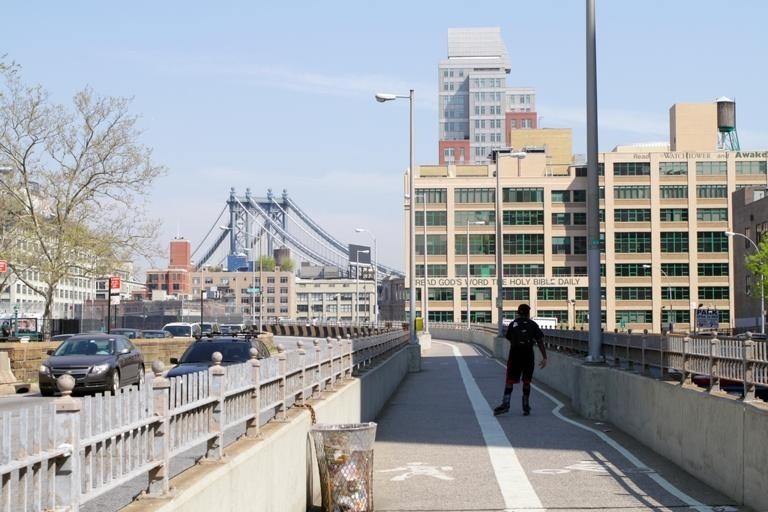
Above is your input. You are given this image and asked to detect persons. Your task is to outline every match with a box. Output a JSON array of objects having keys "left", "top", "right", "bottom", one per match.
[
  {"left": 17, "top": 320, "right": 32, "bottom": 334},
  {"left": 311, "top": 315, "right": 317, "bottom": 327},
  {"left": 493, "top": 303, "right": 547, "bottom": 414},
  {"left": 362, "top": 318, "right": 368, "bottom": 326}
]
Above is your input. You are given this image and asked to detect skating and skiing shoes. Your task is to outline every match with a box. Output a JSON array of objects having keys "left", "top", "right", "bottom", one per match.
[
  {"left": 522, "top": 389, "right": 532, "bottom": 415},
  {"left": 494, "top": 388, "right": 512, "bottom": 416}
]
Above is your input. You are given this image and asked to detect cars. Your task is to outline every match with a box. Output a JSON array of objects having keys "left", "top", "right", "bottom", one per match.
[
  {"left": 36, "top": 331, "right": 146, "bottom": 399},
  {"left": 687, "top": 331, "right": 767, "bottom": 340},
  {"left": 0, "top": 328, "right": 175, "bottom": 342}
]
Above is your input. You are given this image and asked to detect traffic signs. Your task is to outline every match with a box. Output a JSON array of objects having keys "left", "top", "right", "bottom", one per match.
[{"left": 245, "top": 288, "right": 261, "bottom": 293}]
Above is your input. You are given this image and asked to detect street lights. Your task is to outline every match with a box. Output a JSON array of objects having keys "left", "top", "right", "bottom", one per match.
[
  {"left": 565, "top": 298, "right": 577, "bottom": 331},
  {"left": 199, "top": 289, "right": 207, "bottom": 335},
  {"left": 355, "top": 227, "right": 378, "bottom": 328},
  {"left": 355, "top": 249, "right": 370, "bottom": 331},
  {"left": 218, "top": 225, "right": 264, "bottom": 333},
  {"left": 373, "top": 87, "right": 421, "bottom": 371},
  {"left": 641, "top": 263, "right": 672, "bottom": 322},
  {"left": 723, "top": 231, "right": 765, "bottom": 333},
  {"left": 495, "top": 149, "right": 528, "bottom": 334},
  {"left": 235, "top": 245, "right": 255, "bottom": 325},
  {"left": 692, "top": 302, "right": 703, "bottom": 332},
  {"left": 403, "top": 192, "right": 428, "bottom": 335},
  {"left": 465, "top": 219, "right": 486, "bottom": 330}
]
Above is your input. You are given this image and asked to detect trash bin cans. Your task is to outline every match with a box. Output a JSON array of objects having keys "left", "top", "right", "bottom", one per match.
[{"left": 311, "top": 421, "right": 377, "bottom": 512}]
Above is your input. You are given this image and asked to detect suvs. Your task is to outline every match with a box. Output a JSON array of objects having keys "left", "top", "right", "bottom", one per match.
[{"left": 160, "top": 335, "right": 272, "bottom": 380}]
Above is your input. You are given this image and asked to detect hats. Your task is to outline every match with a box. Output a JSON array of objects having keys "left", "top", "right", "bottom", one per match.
[{"left": 518, "top": 303, "right": 531, "bottom": 314}]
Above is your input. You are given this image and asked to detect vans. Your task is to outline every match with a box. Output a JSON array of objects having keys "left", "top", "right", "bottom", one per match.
[{"left": 156, "top": 320, "right": 248, "bottom": 338}]
